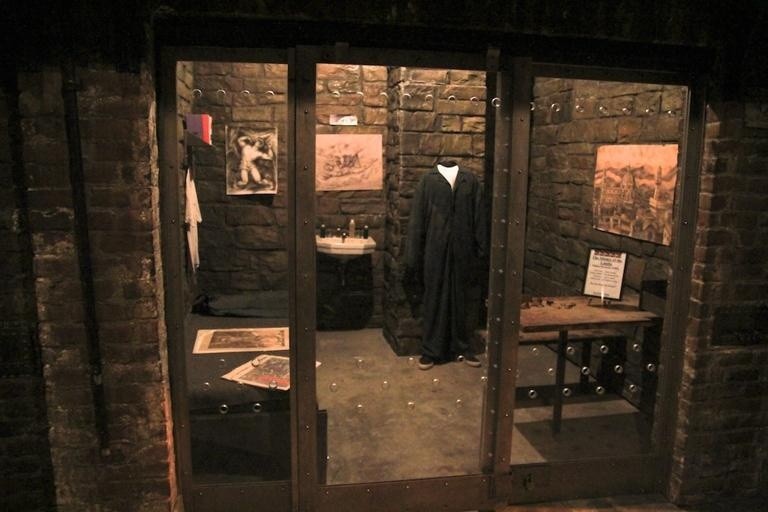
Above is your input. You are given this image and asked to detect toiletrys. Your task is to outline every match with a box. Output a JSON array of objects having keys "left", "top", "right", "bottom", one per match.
[{"left": 348, "top": 218, "right": 356, "bottom": 237}]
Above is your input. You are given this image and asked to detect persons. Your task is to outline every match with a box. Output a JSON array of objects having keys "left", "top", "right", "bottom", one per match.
[
  {"left": 234, "top": 135, "right": 275, "bottom": 189},
  {"left": 404, "top": 160, "right": 486, "bottom": 371}
]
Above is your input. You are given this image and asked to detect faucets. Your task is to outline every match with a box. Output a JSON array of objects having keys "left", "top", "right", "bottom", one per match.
[{"left": 341, "top": 232, "right": 346, "bottom": 242}]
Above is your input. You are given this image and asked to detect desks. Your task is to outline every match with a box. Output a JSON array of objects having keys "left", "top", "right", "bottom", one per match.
[{"left": 484, "top": 290, "right": 669, "bottom": 439}]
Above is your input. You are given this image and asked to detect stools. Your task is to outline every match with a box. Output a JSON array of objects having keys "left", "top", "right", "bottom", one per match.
[
  {"left": 185, "top": 311, "right": 329, "bottom": 481},
  {"left": 480, "top": 329, "right": 625, "bottom": 387}
]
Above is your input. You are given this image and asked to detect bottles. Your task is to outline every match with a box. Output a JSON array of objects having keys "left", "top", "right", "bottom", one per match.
[{"left": 348, "top": 219, "right": 356, "bottom": 238}]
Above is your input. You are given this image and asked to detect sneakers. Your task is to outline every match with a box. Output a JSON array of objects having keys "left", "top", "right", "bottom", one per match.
[
  {"left": 417, "top": 353, "right": 434, "bottom": 370},
  {"left": 464, "top": 355, "right": 481, "bottom": 368}
]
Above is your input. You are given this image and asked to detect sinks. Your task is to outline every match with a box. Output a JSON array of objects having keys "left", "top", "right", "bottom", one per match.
[{"left": 316, "top": 236, "right": 378, "bottom": 257}]
